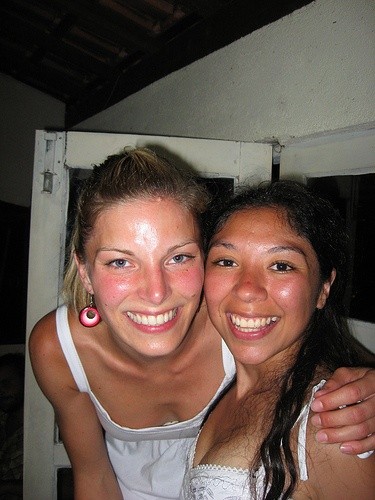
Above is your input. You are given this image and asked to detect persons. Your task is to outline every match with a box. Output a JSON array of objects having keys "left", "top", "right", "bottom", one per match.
[
  {"left": 179, "top": 175, "right": 375, "bottom": 499},
  {"left": 27, "top": 138, "right": 375, "bottom": 500}
]
